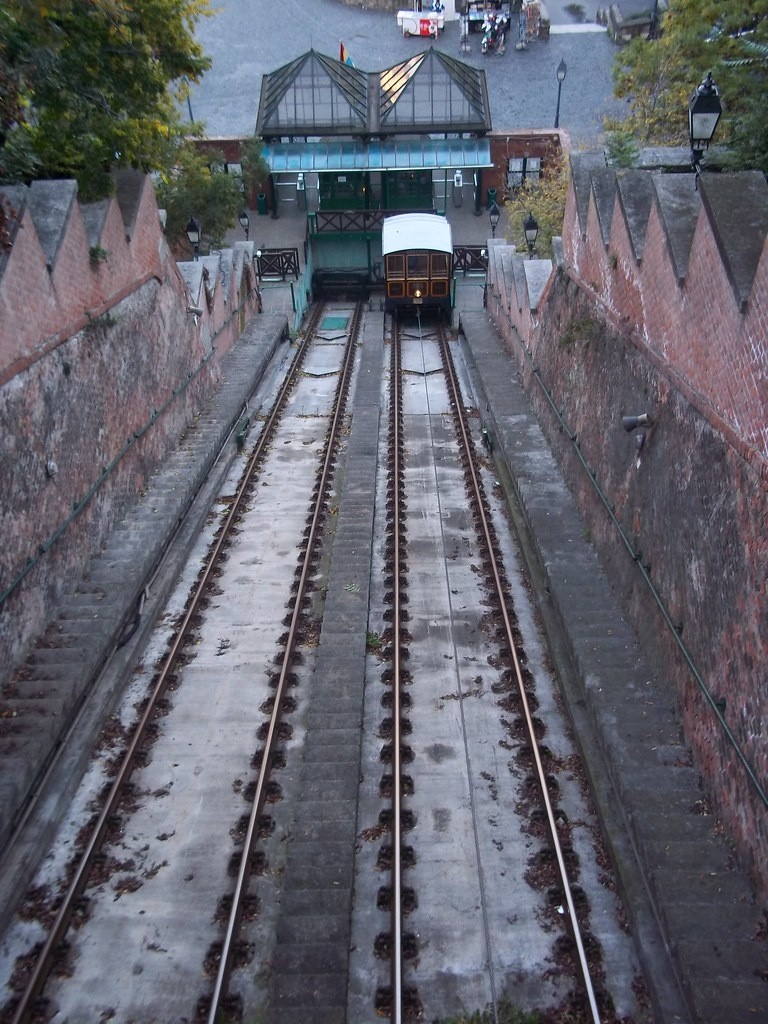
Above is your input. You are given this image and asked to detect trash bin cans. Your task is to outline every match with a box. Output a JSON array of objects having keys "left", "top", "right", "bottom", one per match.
[
  {"left": 487, "top": 188, "right": 497, "bottom": 211},
  {"left": 257, "top": 193, "right": 267, "bottom": 215}
]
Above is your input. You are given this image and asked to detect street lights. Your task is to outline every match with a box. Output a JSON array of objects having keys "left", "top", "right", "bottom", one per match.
[
  {"left": 554, "top": 57, "right": 568, "bottom": 129},
  {"left": 523, "top": 210, "right": 538, "bottom": 260},
  {"left": 240, "top": 210, "right": 249, "bottom": 242},
  {"left": 184, "top": 214, "right": 203, "bottom": 263},
  {"left": 489, "top": 202, "right": 501, "bottom": 238}
]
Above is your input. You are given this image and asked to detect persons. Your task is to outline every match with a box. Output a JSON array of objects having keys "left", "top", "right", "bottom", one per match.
[
  {"left": 432, "top": 0, "right": 442, "bottom": 12},
  {"left": 482, "top": 14, "right": 498, "bottom": 47}
]
